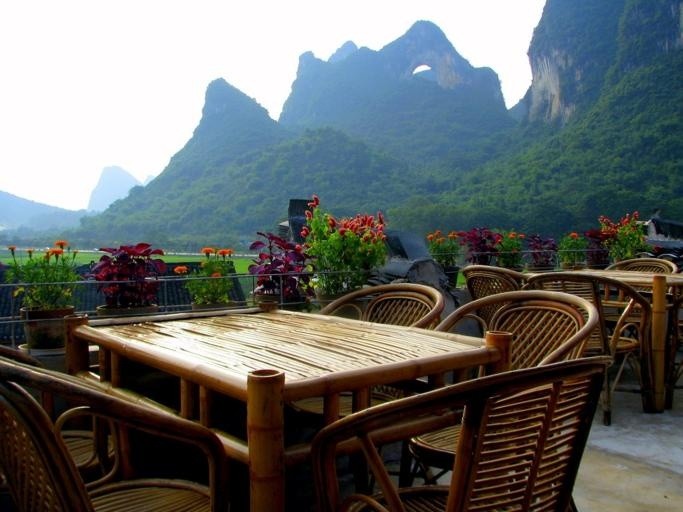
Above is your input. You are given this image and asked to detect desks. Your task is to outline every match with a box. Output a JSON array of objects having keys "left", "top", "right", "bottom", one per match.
[{"left": 70, "top": 301, "right": 518, "bottom": 512}]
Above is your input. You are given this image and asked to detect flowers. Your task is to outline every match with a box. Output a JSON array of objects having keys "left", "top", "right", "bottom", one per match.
[
  {"left": 525, "top": 233, "right": 557, "bottom": 270},
  {"left": 294, "top": 194, "right": 386, "bottom": 295},
  {"left": 599, "top": 210, "right": 663, "bottom": 256},
  {"left": 459, "top": 224, "right": 497, "bottom": 256},
  {"left": 174, "top": 247, "right": 235, "bottom": 303},
  {"left": 9, "top": 240, "right": 86, "bottom": 308},
  {"left": 495, "top": 228, "right": 525, "bottom": 268},
  {"left": 581, "top": 226, "right": 609, "bottom": 265},
  {"left": 558, "top": 230, "right": 588, "bottom": 261},
  {"left": 427, "top": 230, "right": 460, "bottom": 268}
]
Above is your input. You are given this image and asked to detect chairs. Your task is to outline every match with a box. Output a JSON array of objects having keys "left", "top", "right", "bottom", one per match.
[
  {"left": 458, "top": 251, "right": 681, "bottom": 428},
  {"left": 0, "top": 356, "right": 238, "bottom": 510},
  {"left": 308, "top": 351, "right": 619, "bottom": 512},
  {"left": 401, "top": 285, "right": 598, "bottom": 487},
  {"left": 284, "top": 277, "right": 449, "bottom": 458},
  {"left": 0, "top": 344, "right": 128, "bottom": 484}
]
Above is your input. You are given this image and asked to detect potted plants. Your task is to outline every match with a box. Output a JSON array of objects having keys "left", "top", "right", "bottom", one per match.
[
  {"left": 84, "top": 241, "right": 166, "bottom": 314},
  {"left": 249, "top": 227, "right": 307, "bottom": 308}
]
